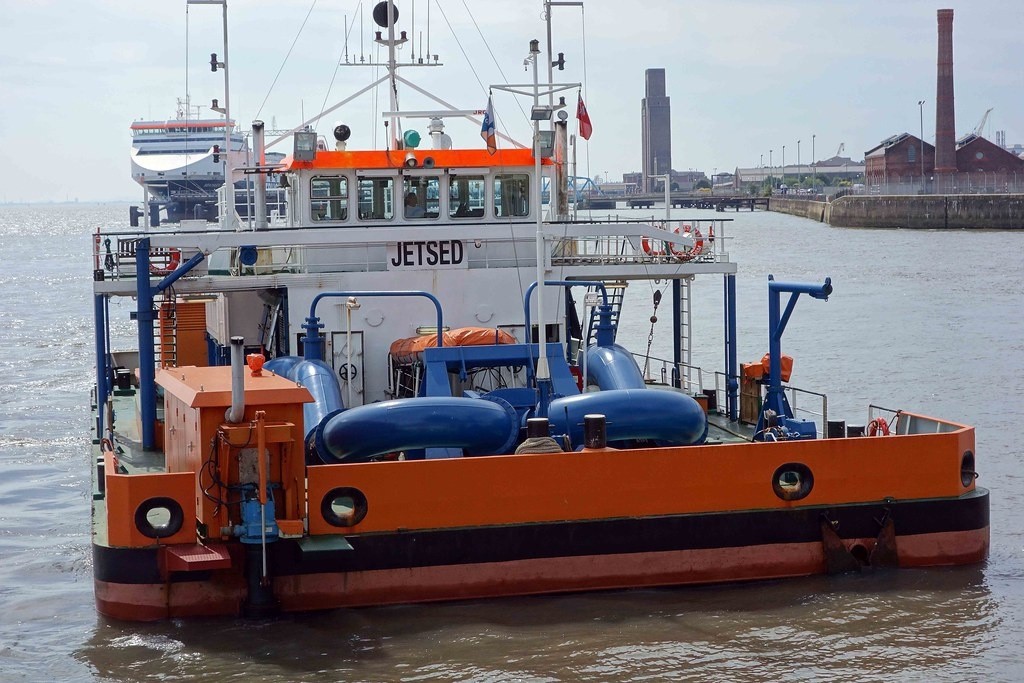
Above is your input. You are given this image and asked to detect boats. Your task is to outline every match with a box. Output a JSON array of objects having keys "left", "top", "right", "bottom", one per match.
[{"left": 88, "top": 1, "right": 992, "bottom": 627}]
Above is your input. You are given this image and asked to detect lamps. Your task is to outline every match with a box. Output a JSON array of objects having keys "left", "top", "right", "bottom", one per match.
[
  {"left": 405, "top": 152, "right": 418, "bottom": 169},
  {"left": 294, "top": 132, "right": 318, "bottom": 163},
  {"left": 532, "top": 131, "right": 555, "bottom": 159},
  {"left": 531, "top": 105, "right": 554, "bottom": 120}
]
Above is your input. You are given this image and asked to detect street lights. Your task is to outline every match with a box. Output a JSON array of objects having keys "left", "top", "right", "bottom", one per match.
[
  {"left": 917, "top": 100, "right": 926, "bottom": 193},
  {"left": 761, "top": 155, "right": 763, "bottom": 169},
  {"left": 812, "top": 135, "right": 816, "bottom": 196},
  {"left": 783, "top": 146, "right": 785, "bottom": 186},
  {"left": 770, "top": 150, "right": 772, "bottom": 185},
  {"left": 605, "top": 171, "right": 608, "bottom": 185},
  {"left": 797, "top": 140, "right": 800, "bottom": 190}
]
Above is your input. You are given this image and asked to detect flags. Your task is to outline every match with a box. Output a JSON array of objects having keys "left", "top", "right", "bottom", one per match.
[
  {"left": 480, "top": 95, "right": 497, "bottom": 156},
  {"left": 576, "top": 92, "right": 592, "bottom": 140}
]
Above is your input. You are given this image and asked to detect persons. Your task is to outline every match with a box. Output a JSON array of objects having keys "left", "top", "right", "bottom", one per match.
[{"left": 406, "top": 192, "right": 424, "bottom": 217}]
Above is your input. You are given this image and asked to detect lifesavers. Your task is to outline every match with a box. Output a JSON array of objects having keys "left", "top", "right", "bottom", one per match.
[
  {"left": 668, "top": 224, "right": 703, "bottom": 261},
  {"left": 147, "top": 246, "right": 181, "bottom": 278},
  {"left": 642, "top": 224, "right": 672, "bottom": 257},
  {"left": 868, "top": 415, "right": 889, "bottom": 438}
]
